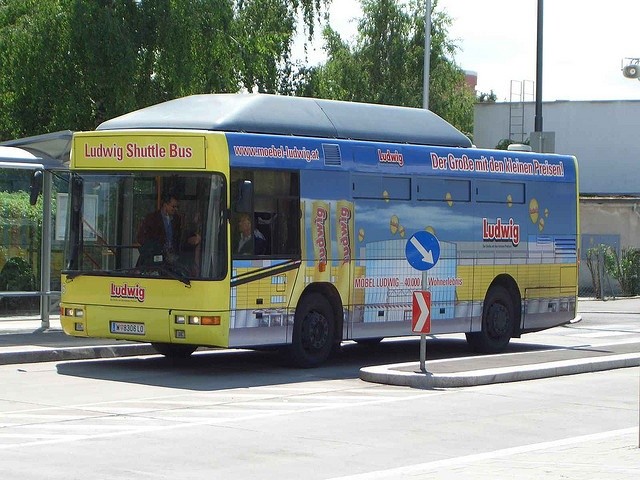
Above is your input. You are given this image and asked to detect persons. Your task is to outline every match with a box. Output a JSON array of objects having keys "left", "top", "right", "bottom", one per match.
[
  {"left": 235, "top": 214, "right": 267, "bottom": 255},
  {"left": 135, "top": 192, "right": 177, "bottom": 271},
  {"left": 180, "top": 209, "right": 202, "bottom": 275}
]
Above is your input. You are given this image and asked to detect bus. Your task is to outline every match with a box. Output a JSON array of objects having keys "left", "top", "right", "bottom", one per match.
[{"left": 30, "top": 92, "right": 581, "bottom": 368}]
